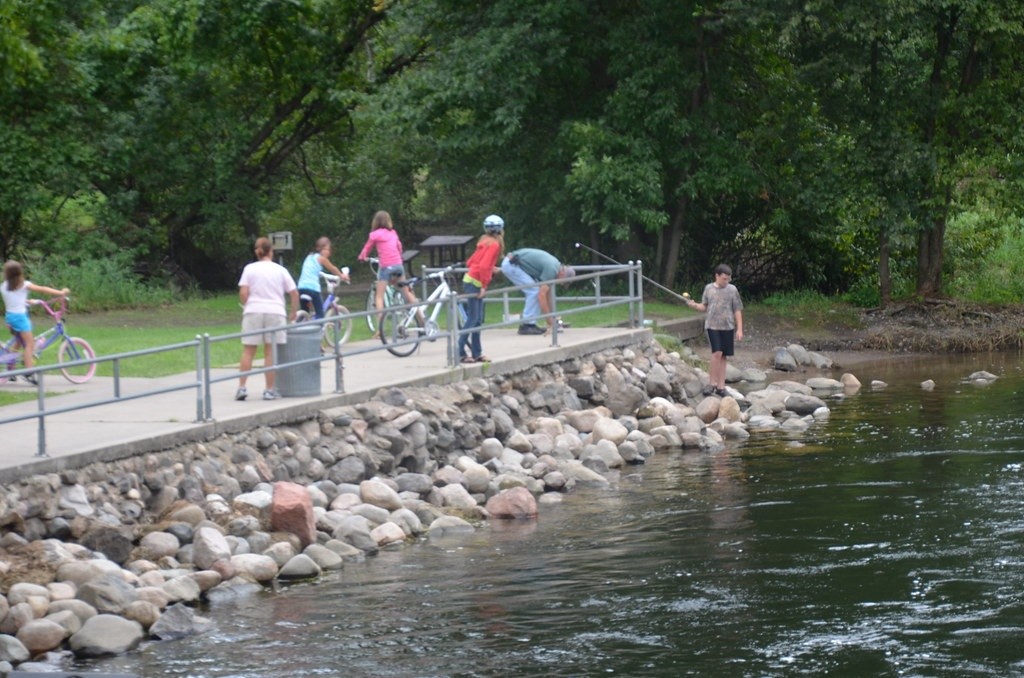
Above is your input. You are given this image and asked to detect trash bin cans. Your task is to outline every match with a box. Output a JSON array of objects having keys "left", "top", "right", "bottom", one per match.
[{"left": 274, "top": 325, "right": 321, "bottom": 396}]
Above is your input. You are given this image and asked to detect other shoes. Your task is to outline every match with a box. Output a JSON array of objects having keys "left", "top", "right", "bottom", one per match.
[
  {"left": 518, "top": 324, "right": 543, "bottom": 335},
  {"left": 704, "top": 385, "right": 718, "bottom": 395},
  {"left": 460, "top": 356, "right": 475, "bottom": 363},
  {"left": 25, "top": 374, "right": 38, "bottom": 386},
  {"left": 478, "top": 355, "right": 491, "bottom": 362},
  {"left": 234, "top": 387, "right": 247, "bottom": 401},
  {"left": 7, "top": 368, "right": 17, "bottom": 381},
  {"left": 719, "top": 388, "right": 733, "bottom": 397},
  {"left": 263, "top": 390, "right": 280, "bottom": 400}
]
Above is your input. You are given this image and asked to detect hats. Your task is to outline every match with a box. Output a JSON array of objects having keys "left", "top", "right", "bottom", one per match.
[{"left": 564, "top": 269, "right": 576, "bottom": 290}]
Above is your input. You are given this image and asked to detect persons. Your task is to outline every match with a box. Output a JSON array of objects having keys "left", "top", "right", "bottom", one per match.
[
  {"left": 235, "top": 237, "right": 299, "bottom": 401},
  {"left": 0, "top": 260, "right": 70, "bottom": 387},
  {"left": 689, "top": 263, "right": 743, "bottom": 396},
  {"left": 297, "top": 237, "right": 349, "bottom": 328},
  {"left": 501, "top": 248, "right": 576, "bottom": 335},
  {"left": 358, "top": 210, "right": 425, "bottom": 339},
  {"left": 458, "top": 215, "right": 505, "bottom": 363}
]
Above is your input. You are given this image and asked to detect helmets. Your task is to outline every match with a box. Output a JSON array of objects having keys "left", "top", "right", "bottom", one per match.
[{"left": 484, "top": 215, "right": 504, "bottom": 227}]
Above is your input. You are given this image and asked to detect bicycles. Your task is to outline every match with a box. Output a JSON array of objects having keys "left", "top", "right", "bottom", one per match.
[
  {"left": 0, "top": 291, "right": 99, "bottom": 389},
  {"left": 377, "top": 260, "right": 469, "bottom": 358},
  {"left": 358, "top": 256, "right": 413, "bottom": 333},
  {"left": 293, "top": 267, "right": 355, "bottom": 352}
]
